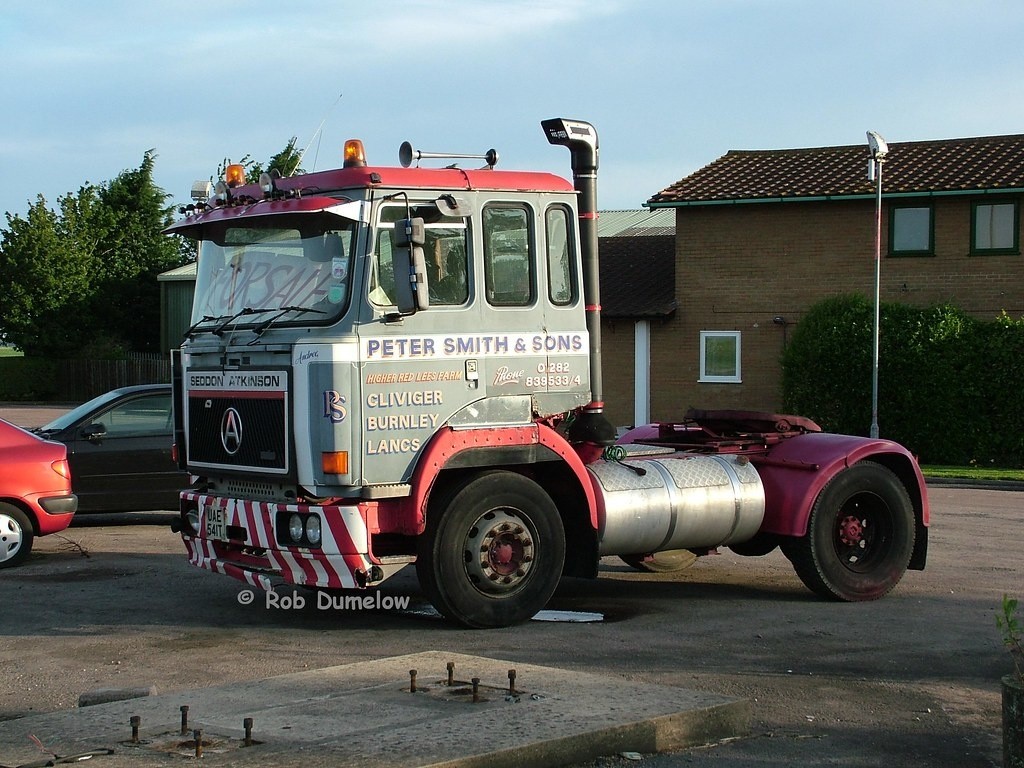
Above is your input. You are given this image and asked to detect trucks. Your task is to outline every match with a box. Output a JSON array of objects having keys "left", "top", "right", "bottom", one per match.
[{"left": 164, "top": 115, "right": 932, "bottom": 631}]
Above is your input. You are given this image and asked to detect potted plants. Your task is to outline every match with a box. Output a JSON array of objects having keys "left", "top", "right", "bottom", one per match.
[{"left": 992, "top": 591, "right": 1024, "bottom": 768}]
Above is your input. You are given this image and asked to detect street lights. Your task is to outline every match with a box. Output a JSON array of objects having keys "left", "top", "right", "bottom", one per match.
[{"left": 864, "top": 130, "right": 890, "bottom": 439}]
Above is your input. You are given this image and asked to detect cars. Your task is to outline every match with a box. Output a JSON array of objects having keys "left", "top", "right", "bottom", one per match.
[
  {"left": 16, "top": 381, "right": 206, "bottom": 529},
  {"left": 0, "top": 416, "right": 79, "bottom": 571}
]
